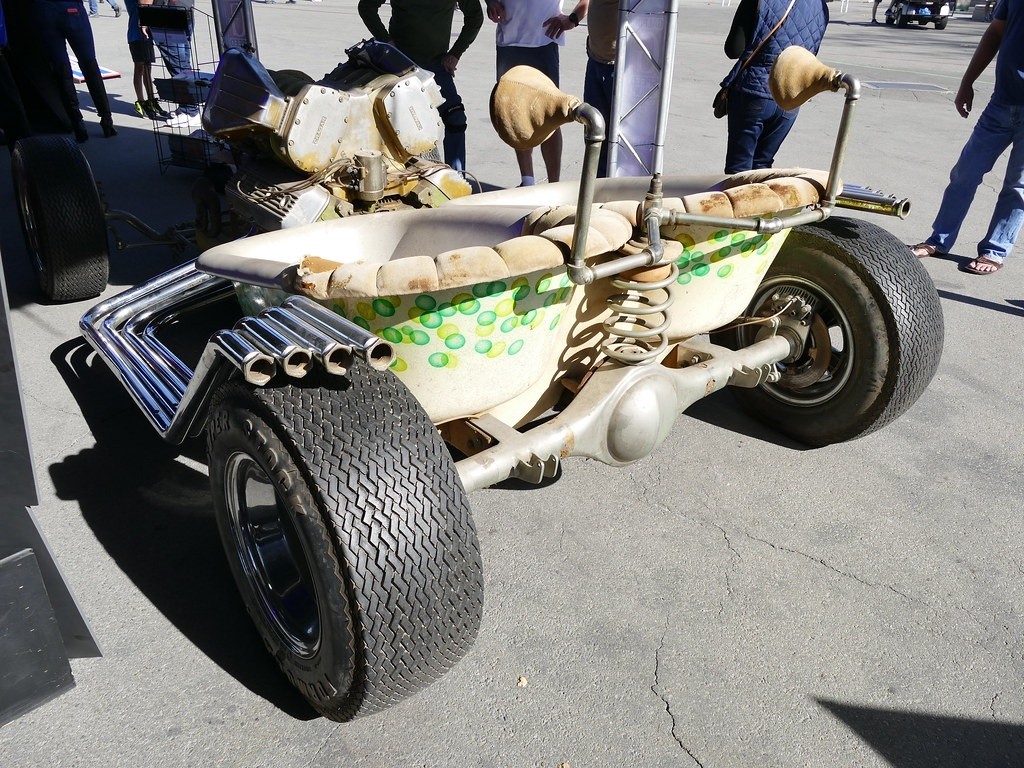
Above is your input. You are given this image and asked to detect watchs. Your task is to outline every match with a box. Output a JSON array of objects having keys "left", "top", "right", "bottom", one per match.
[{"left": 568, "top": 13, "right": 578, "bottom": 28}]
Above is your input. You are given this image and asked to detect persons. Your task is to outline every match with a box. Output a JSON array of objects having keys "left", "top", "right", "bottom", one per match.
[
  {"left": 871, "top": 0, "right": 883, "bottom": 25},
  {"left": 485, "top": 0, "right": 590, "bottom": 191},
  {"left": 123, "top": 0, "right": 171, "bottom": 119},
  {"left": 358, "top": 0, "right": 484, "bottom": 180},
  {"left": 905, "top": 0, "right": 1024, "bottom": 275},
  {"left": 582, "top": 0, "right": 618, "bottom": 178},
  {"left": 138, "top": 0, "right": 202, "bottom": 128},
  {"left": 0, "top": 0, "right": 34, "bottom": 158},
  {"left": 981, "top": 0, "right": 998, "bottom": 22},
  {"left": 724, "top": 0, "right": 829, "bottom": 174},
  {"left": 28, "top": 0, "right": 121, "bottom": 144}
]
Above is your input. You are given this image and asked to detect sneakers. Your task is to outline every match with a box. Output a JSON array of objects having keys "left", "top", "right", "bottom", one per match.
[
  {"left": 170, "top": 110, "right": 180, "bottom": 119},
  {"left": 167, "top": 113, "right": 202, "bottom": 128}
]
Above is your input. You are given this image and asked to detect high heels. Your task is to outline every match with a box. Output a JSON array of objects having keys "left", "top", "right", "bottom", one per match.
[
  {"left": 100, "top": 117, "right": 117, "bottom": 137},
  {"left": 75, "top": 130, "right": 88, "bottom": 142}
]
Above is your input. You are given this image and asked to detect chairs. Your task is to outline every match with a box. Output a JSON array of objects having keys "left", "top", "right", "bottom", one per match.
[
  {"left": 447, "top": 167, "right": 843, "bottom": 343},
  {"left": 193, "top": 205, "right": 626, "bottom": 428}
]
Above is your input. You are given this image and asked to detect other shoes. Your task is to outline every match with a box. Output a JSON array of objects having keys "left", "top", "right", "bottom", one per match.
[
  {"left": 132, "top": 100, "right": 156, "bottom": 120},
  {"left": 148, "top": 97, "right": 170, "bottom": 120}
]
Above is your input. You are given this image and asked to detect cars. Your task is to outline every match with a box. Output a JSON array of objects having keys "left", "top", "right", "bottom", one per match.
[{"left": 884, "top": 0, "right": 955, "bottom": 30}]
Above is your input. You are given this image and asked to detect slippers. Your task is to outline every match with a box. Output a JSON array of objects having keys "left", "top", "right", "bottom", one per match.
[
  {"left": 964, "top": 256, "right": 1003, "bottom": 275},
  {"left": 907, "top": 243, "right": 948, "bottom": 258}
]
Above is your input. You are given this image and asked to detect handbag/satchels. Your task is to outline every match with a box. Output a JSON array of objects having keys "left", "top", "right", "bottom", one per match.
[{"left": 713, "top": 87, "right": 728, "bottom": 119}]
